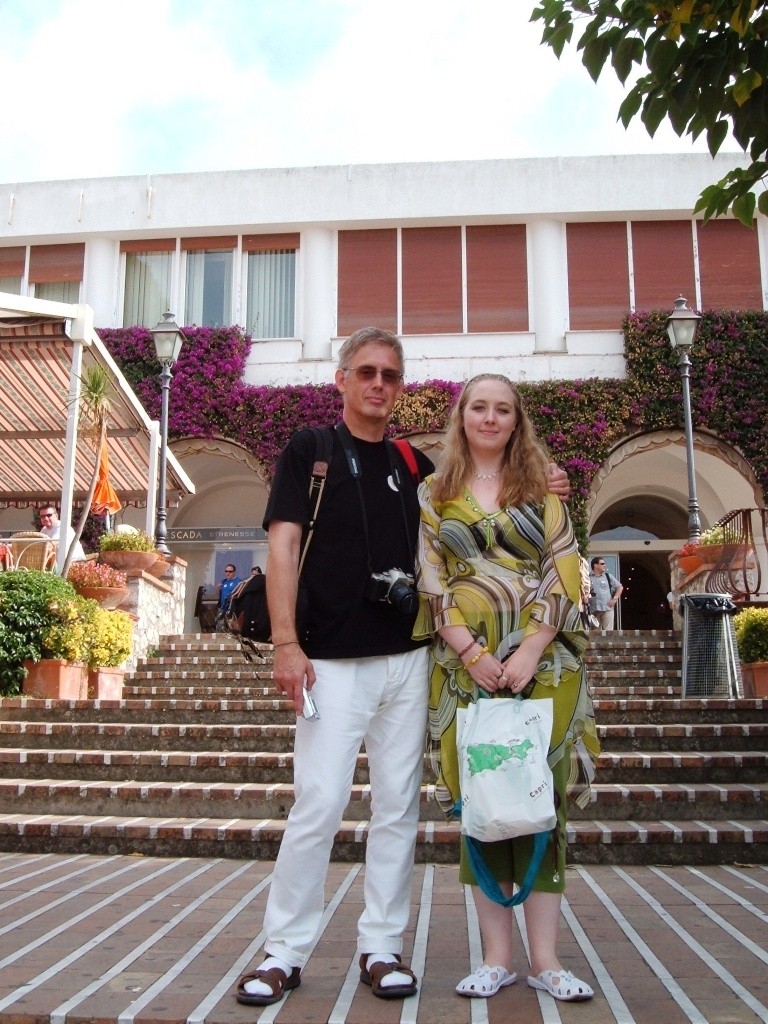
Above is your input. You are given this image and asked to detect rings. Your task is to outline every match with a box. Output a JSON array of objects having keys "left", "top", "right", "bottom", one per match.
[
  {"left": 513, "top": 682, "right": 518, "bottom": 687},
  {"left": 282, "top": 691, "right": 287, "bottom": 695},
  {"left": 501, "top": 676, "right": 508, "bottom": 682}
]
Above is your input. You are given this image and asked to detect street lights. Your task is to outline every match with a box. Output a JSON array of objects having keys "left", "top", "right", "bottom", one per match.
[
  {"left": 666, "top": 292, "right": 702, "bottom": 550},
  {"left": 150, "top": 308, "right": 189, "bottom": 557}
]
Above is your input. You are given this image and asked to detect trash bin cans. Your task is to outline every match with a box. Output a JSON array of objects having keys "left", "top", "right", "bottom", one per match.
[{"left": 678, "top": 593, "right": 744, "bottom": 700}]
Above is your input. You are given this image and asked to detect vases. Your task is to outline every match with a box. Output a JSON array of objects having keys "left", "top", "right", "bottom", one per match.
[
  {"left": 741, "top": 664, "right": 768, "bottom": 699},
  {"left": 678, "top": 555, "right": 704, "bottom": 575},
  {"left": 695, "top": 545, "right": 753, "bottom": 565}
]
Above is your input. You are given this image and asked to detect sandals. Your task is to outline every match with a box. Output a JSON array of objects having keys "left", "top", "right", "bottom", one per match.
[
  {"left": 358, "top": 951, "right": 420, "bottom": 997},
  {"left": 236, "top": 953, "right": 302, "bottom": 1007}
]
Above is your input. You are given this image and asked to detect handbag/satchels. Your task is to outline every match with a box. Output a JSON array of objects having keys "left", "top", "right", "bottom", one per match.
[
  {"left": 453, "top": 687, "right": 558, "bottom": 844},
  {"left": 222, "top": 571, "right": 280, "bottom": 662}
]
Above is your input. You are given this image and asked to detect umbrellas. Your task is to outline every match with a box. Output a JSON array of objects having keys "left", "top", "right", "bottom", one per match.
[{"left": 90, "top": 422, "right": 121, "bottom": 531}]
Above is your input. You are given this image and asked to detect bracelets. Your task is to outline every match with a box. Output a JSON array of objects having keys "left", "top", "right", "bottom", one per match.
[
  {"left": 463, "top": 647, "right": 489, "bottom": 670},
  {"left": 274, "top": 641, "right": 299, "bottom": 648},
  {"left": 459, "top": 640, "right": 476, "bottom": 658}
]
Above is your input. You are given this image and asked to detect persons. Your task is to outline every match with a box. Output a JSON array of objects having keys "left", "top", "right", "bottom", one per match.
[
  {"left": 216, "top": 564, "right": 242, "bottom": 633},
  {"left": 40, "top": 504, "right": 85, "bottom": 564},
  {"left": 251, "top": 566, "right": 262, "bottom": 575},
  {"left": 412, "top": 374, "right": 599, "bottom": 999},
  {"left": 235, "top": 328, "right": 570, "bottom": 1003},
  {"left": 589, "top": 556, "right": 623, "bottom": 630}
]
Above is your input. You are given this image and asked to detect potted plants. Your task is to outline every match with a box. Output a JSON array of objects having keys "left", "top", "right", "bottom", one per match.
[
  {"left": 99, "top": 530, "right": 158, "bottom": 570},
  {"left": 80, "top": 605, "right": 133, "bottom": 699},
  {"left": 67, "top": 560, "right": 130, "bottom": 610},
  {"left": 22, "top": 598, "right": 89, "bottom": 700},
  {"left": 146, "top": 549, "right": 171, "bottom": 578}
]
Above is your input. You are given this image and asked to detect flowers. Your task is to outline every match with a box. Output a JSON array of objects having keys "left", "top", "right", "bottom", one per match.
[
  {"left": 734, "top": 605, "right": 768, "bottom": 663},
  {"left": 676, "top": 543, "right": 700, "bottom": 556},
  {"left": 700, "top": 526, "right": 745, "bottom": 543}
]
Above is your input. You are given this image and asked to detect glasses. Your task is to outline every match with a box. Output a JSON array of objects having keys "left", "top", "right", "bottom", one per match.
[
  {"left": 40, "top": 512, "right": 57, "bottom": 518},
  {"left": 344, "top": 365, "right": 404, "bottom": 385},
  {"left": 597, "top": 563, "right": 606, "bottom": 566},
  {"left": 224, "top": 569, "right": 236, "bottom": 573}
]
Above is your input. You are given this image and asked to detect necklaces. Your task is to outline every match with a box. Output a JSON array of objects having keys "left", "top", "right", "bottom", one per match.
[{"left": 474, "top": 470, "right": 500, "bottom": 479}]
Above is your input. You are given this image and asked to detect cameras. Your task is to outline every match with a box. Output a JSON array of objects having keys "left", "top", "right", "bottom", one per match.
[{"left": 302, "top": 686, "right": 320, "bottom": 722}]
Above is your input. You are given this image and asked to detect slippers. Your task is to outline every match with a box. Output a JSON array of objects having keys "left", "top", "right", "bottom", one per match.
[
  {"left": 527, "top": 969, "right": 596, "bottom": 1002},
  {"left": 455, "top": 965, "right": 519, "bottom": 998}
]
